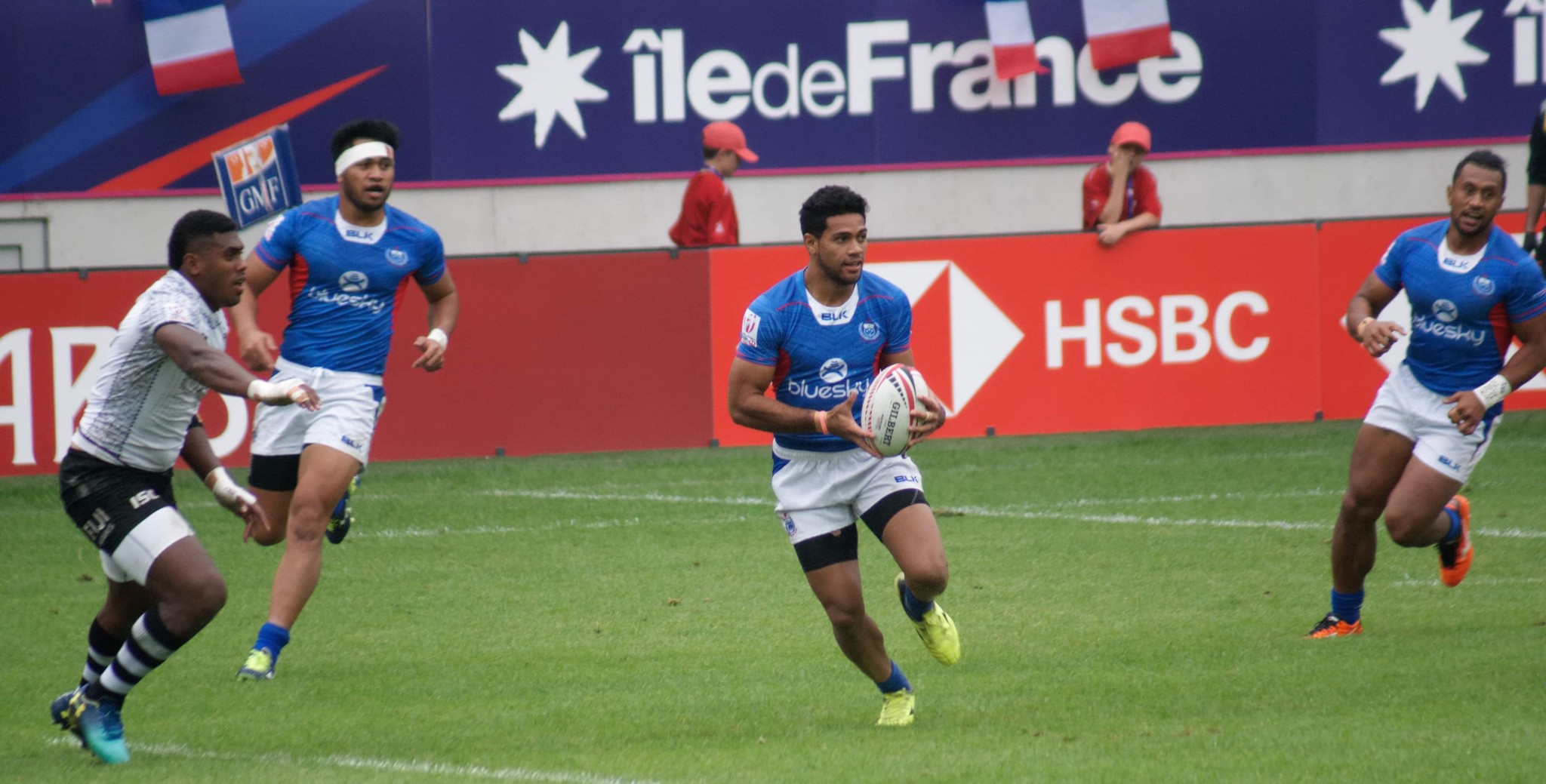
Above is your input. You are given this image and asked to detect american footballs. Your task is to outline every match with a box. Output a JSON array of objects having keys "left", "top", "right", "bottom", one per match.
[{"left": 858, "top": 362, "right": 928, "bottom": 455}]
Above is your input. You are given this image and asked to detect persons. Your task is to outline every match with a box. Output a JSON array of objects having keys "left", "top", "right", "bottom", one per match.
[
  {"left": 227, "top": 119, "right": 458, "bottom": 681},
  {"left": 1522, "top": 109, "right": 1546, "bottom": 279},
  {"left": 668, "top": 122, "right": 760, "bottom": 248},
  {"left": 728, "top": 185, "right": 961, "bottom": 730},
  {"left": 1309, "top": 152, "right": 1546, "bottom": 638},
  {"left": 49, "top": 210, "right": 321, "bottom": 763},
  {"left": 1082, "top": 120, "right": 1163, "bottom": 244}
]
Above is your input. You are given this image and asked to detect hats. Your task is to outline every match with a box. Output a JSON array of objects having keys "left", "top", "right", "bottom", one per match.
[
  {"left": 703, "top": 121, "right": 758, "bottom": 163},
  {"left": 1111, "top": 122, "right": 1151, "bottom": 152}
]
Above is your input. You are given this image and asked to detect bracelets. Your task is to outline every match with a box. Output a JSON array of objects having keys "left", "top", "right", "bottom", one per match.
[
  {"left": 815, "top": 410, "right": 829, "bottom": 436},
  {"left": 426, "top": 328, "right": 448, "bottom": 351},
  {"left": 1357, "top": 316, "right": 1375, "bottom": 336},
  {"left": 1473, "top": 374, "right": 1511, "bottom": 410}
]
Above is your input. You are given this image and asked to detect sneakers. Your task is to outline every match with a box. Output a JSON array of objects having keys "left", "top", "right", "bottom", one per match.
[
  {"left": 71, "top": 684, "right": 130, "bottom": 764},
  {"left": 875, "top": 687, "right": 916, "bottom": 727},
  {"left": 325, "top": 475, "right": 360, "bottom": 544},
  {"left": 50, "top": 691, "right": 86, "bottom": 745},
  {"left": 1436, "top": 495, "right": 1474, "bottom": 587},
  {"left": 1304, "top": 611, "right": 1362, "bottom": 638},
  {"left": 895, "top": 572, "right": 960, "bottom": 666},
  {"left": 235, "top": 646, "right": 275, "bottom": 681}
]
